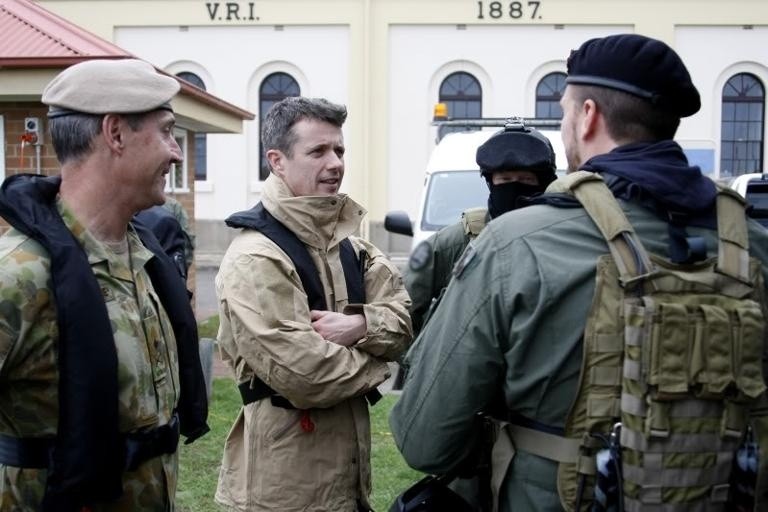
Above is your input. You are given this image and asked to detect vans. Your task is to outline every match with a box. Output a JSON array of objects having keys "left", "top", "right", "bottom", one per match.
[{"left": 383, "top": 119, "right": 571, "bottom": 253}]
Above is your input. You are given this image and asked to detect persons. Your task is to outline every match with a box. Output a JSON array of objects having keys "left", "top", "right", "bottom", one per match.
[
  {"left": 393, "top": 123, "right": 559, "bottom": 390},
  {"left": 1, "top": 58, "right": 211, "bottom": 512},
  {"left": 159, "top": 195, "right": 197, "bottom": 267},
  {"left": 133, "top": 204, "right": 193, "bottom": 287},
  {"left": 213, "top": 95, "right": 416, "bottom": 511},
  {"left": 389, "top": 33, "right": 768, "bottom": 511}
]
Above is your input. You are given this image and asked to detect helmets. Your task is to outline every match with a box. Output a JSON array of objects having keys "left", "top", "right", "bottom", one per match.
[{"left": 478, "top": 120, "right": 556, "bottom": 174}]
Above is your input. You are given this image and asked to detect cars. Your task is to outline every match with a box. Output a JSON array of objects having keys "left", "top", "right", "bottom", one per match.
[{"left": 728, "top": 172, "right": 768, "bottom": 229}]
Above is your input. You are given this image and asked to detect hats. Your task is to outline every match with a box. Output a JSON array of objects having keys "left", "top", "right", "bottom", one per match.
[
  {"left": 566, "top": 33, "right": 702, "bottom": 118},
  {"left": 42, "top": 57, "right": 180, "bottom": 116}
]
